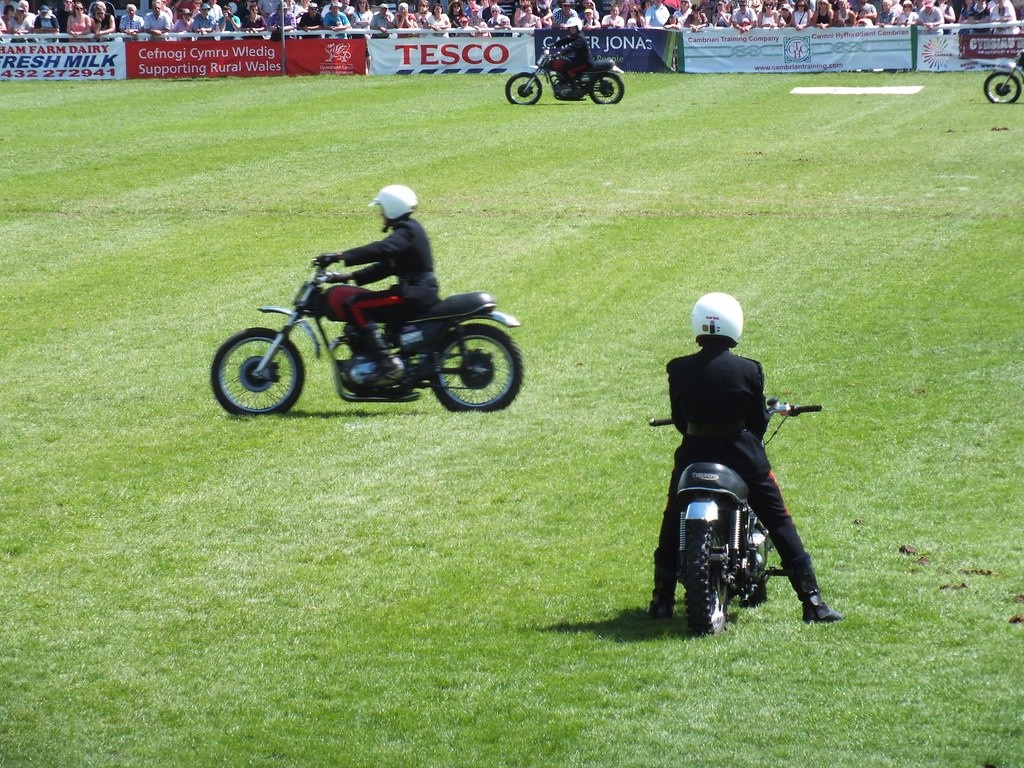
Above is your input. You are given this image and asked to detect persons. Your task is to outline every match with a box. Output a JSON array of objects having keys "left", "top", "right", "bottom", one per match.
[
  {"left": 312, "top": 182, "right": 442, "bottom": 380},
  {"left": 642, "top": 292, "right": 845, "bottom": 624},
  {"left": 0, "top": 1, "right": 1024, "bottom": 43},
  {"left": 546, "top": 17, "right": 593, "bottom": 90}
]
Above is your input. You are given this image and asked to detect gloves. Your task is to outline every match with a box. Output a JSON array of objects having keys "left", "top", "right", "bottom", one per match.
[
  {"left": 325, "top": 273, "right": 347, "bottom": 283},
  {"left": 551, "top": 53, "right": 558, "bottom": 59},
  {"left": 314, "top": 252, "right": 338, "bottom": 269}
]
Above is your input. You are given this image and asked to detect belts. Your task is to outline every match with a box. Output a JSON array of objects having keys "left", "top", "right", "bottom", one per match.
[{"left": 686, "top": 422, "right": 749, "bottom": 436}]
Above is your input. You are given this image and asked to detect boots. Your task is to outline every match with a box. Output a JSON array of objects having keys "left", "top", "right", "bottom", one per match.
[
  {"left": 357, "top": 329, "right": 404, "bottom": 384},
  {"left": 784, "top": 563, "right": 841, "bottom": 622},
  {"left": 648, "top": 549, "right": 679, "bottom": 618}
]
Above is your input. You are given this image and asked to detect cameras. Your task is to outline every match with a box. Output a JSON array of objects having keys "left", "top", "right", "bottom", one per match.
[{"left": 227, "top": 12, "right": 234, "bottom": 17}]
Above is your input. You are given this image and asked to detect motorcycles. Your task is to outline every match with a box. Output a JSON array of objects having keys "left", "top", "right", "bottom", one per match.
[
  {"left": 505, "top": 47, "right": 625, "bottom": 105},
  {"left": 983, "top": 50, "right": 1024, "bottom": 105},
  {"left": 211, "top": 255, "right": 524, "bottom": 418},
  {"left": 649, "top": 397, "right": 822, "bottom": 635}
]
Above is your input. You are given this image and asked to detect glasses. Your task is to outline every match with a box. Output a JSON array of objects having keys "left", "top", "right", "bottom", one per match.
[
  {"left": 250, "top": 9, "right": 258, "bottom": 11},
  {"left": 65, "top": 1, "right": 72, "bottom": 3},
  {"left": 74, "top": 8, "right": 83, "bottom": 10},
  {"left": 154, "top": 7, "right": 161, "bottom": 9}
]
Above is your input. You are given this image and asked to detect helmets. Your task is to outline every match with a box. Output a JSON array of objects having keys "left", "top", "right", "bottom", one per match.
[
  {"left": 368, "top": 185, "right": 419, "bottom": 220},
  {"left": 565, "top": 16, "right": 583, "bottom": 32},
  {"left": 691, "top": 292, "right": 744, "bottom": 348}
]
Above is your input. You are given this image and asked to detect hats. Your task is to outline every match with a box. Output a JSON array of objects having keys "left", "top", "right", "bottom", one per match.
[
  {"left": 198, "top": 3, "right": 210, "bottom": 10},
  {"left": 329, "top": 2, "right": 342, "bottom": 10},
  {"left": 40, "top": 5, "right": 48, "bottom": 12},
  {"left": 181, "top": 8, "right": 192, "bottom": 16},
  {"left": 16, "top": 7, "right": 25, "bottom": 12},
  {"left": 375, "top": 0, "right": 935, "bottom": 25},
  {"left": 276, "top": 3, "right": 289, "bottom": 9},
  {"left": 308, "top": 3, "right": 318, "bottom": 7},
  {"left": 448, "top": 0, "right": 461, "bottom": 9}
]
